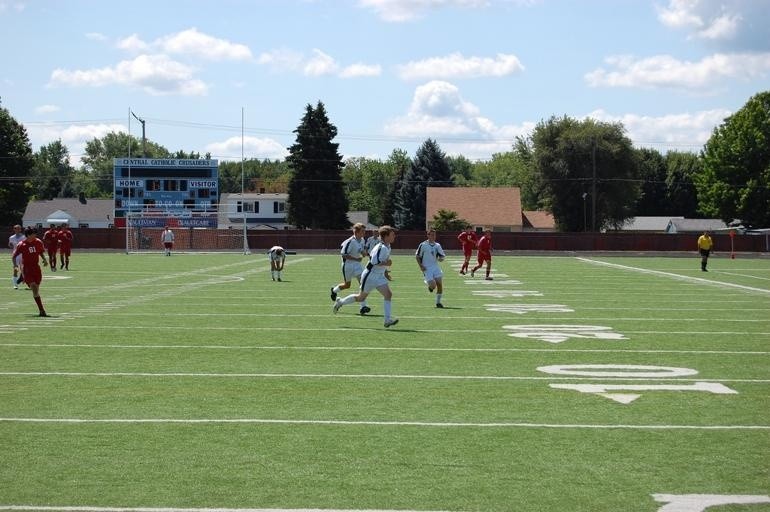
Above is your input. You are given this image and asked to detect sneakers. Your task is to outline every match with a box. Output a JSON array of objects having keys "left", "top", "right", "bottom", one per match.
[
  {"left": 50, "top": 264, "right": 69, "bottom": 272},
  {"left": 39, "top": 312, "right": 46, "bottom": 317},
  {"left": 460, "top": 270, "right": 465, "bottom": 275},
  {"left": 24, "top": 287, "right": 32, "bottom": 290},
  {"left": 16, "top": 273, "right": 23, "bottom": 284},
  {"left": 471, "top": 269, "right": 474, "bottom": 277},
  {"left": 435, "top": 303, "right": 444, "bottom": 308},
  {"left": 485, "top": 276, "right": 494, "bottom": 280},
  {"left": 330, "top": 287, "right": 337, "bottom": 301},
  {"left": 14, "top": 286, "right": 18, "bottom": 289},
  {"left": 384, "top": 318, "right": 399, "bottom": 329},
  {"left": 429, "top": 287, "right": 433, "bottom": 292},
  {"left": 272, "top": 277, "right": 282, "bottom": 282},
  {"left": 332, "top": 297, "right": 342, "bottom": 315},
  {"left": 359, "top": 307, "right": 371, "bottom": 316}
]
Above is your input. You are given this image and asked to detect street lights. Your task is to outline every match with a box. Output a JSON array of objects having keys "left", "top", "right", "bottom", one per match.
[{"left": 582, "top": 192, "right": 588, "bottom": 232}]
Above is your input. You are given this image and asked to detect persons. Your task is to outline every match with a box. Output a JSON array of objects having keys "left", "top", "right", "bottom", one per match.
[
  {"left": 365, "top": 230, "right": 380, "bottom": 256},
  {"left": 331, "top": 223, "right": 371, "bottom": 315},
  {"left": 267, "top": 245, "right": 285, "bottom": 282},
  {"left": 457, "top": 226, "right": 477, "bottom": 275},
  {"left": 415, "top": 230, "right": 445, "bottom": 308},
  {"left": 471, "top": 230, "right": 493, "bottom": 280},
  {"left": 333, "top": 226, "right": 399, "bottom": 327},
  {"left": 697, "top": 231, "right": 713, "bottom": 272},
  {"left": 161, "top": 226, "right": 174, "bottom": 256},
  {"left": 8, "top": 223, "right": 75, "bottom": 317}
]
